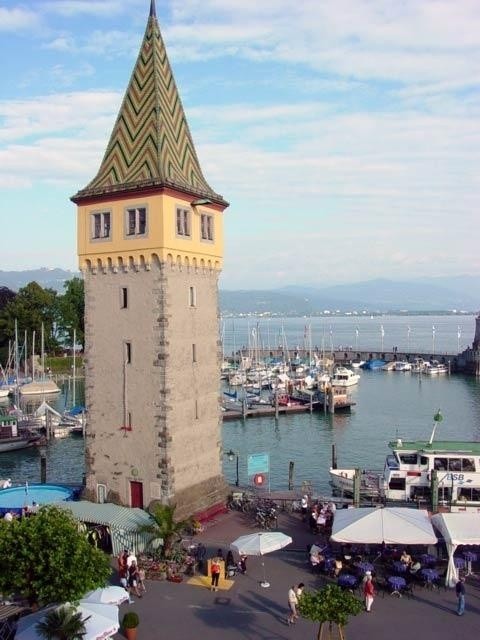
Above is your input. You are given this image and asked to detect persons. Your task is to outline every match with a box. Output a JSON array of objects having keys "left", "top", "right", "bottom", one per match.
[
  {"left": 284, "top": 585, "right": 299, "bottom": 627},
  {"left": 456, "top": 577, "right": 466, "bottom": 615},
  {"left": 294, "top": 582, "right": 305, "bottom": 618},
  {"left": 118, "top": 549, "right": 146, "bottom": 605},
  {"left": 198, "top": 542, "right": 248, "bottom": 592},
  {"left": 300, "top": 493, "right": 337, "bottom": 535},
  {"left": 308, "top": 544, "right": 421, "bottom": 613}
]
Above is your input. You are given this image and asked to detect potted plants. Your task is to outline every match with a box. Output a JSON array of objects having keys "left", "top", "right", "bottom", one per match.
[{"left": 123, "top": 612, "right": 139, "bottom": 640}]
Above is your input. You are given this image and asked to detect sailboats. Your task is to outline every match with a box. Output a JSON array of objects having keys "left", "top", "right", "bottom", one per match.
[
  {"left": 350, "top": 322, "right": 467, "bottom": 376},
  {"left": 0, "top": 318, "right": 87, "bottom": 451},
  {"left": 222, "top": 317, "right": 360, "bottom": 418}
]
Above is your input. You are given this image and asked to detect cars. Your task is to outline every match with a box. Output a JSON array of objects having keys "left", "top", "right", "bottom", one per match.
[{"left": 175, "top": 539, "right": 206, "bottom": 557}]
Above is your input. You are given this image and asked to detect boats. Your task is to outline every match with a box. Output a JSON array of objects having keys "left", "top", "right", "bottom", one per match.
[{"left": 328, "top": 409, "right": 480, "bottom": 506}]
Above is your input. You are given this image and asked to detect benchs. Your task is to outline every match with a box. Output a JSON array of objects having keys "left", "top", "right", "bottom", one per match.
[{"left": 193, "top": 503, "right": 230, "bottom": 529}]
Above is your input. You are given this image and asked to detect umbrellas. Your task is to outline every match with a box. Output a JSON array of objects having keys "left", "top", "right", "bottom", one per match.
[
  {"left": 83, "top": 584, "right": 129, "bottom": 605},
  {"left": 230, "top": 531, "right": 293, "bottom": 583},
  {"left": 14, "top": 598, "right": 120, "bottom": 640}
]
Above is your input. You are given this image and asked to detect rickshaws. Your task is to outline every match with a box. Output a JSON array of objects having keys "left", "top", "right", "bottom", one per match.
[{"left": 126, "top": 551, "right": 151, "bottom": 599}]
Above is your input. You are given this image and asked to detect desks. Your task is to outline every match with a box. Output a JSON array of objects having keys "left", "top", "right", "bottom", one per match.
[{"left": 339, "top": 544, "right": 477, "bottom": 599}]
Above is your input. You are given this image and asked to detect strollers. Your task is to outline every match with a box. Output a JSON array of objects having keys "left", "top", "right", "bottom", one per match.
[{"left": 233, "top": 555, "right": 247, "bottom": 577}]
